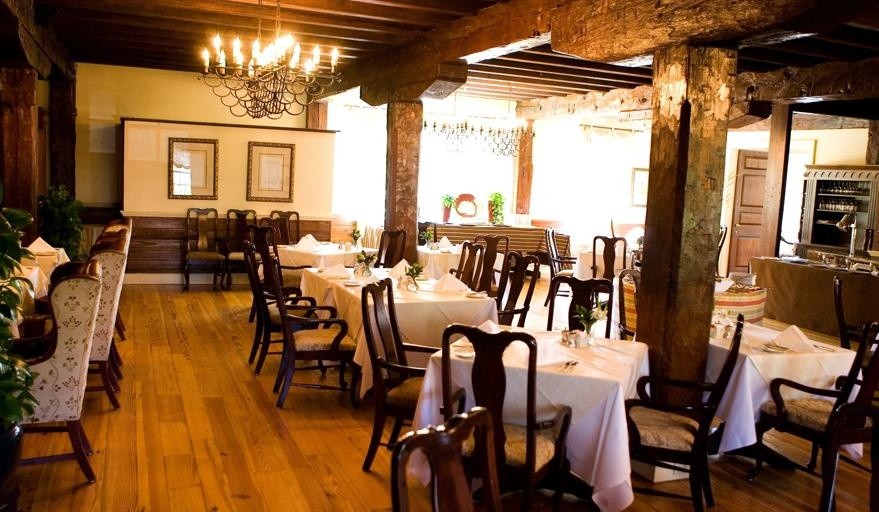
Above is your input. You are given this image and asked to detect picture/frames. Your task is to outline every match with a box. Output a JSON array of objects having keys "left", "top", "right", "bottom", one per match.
[
  {"left": 246, "top": 141, "right": 296, "bottom": 202},
  {"left": 168, "top": 135, "right": 218, "bottom": 200}
]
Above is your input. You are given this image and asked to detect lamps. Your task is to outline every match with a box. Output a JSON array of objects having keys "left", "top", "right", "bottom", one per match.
[{"left": 198, "top": 0, "right": 343, "bottom": 121}]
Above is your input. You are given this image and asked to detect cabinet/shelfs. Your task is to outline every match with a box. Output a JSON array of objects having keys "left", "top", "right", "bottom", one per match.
[{"left": 795, "top": 164, "right": 879, "bottom": 261}]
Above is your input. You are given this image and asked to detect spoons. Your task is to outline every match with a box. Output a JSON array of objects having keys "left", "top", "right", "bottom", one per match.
[{"left": 563, "top": 360, "right": 578, "bottom": 373}]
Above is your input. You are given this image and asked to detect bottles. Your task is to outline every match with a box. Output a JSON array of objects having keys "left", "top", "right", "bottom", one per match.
[
  {"left": 345, "top": 242, "right": 352, "bottom": 251},
  {"left": 560, "top": 327, "right": 568, "bottom": 345},
  {"left": 568, "top": 332, "right": 577, "bottom": 347},
  {"left": 818, "top": 181, "right": 858, "bottom": 213},
  {"left": 709, "top": 321, "right": 733, "bottom": 340}
]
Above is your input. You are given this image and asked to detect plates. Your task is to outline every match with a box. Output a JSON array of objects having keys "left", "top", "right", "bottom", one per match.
[
  {"left": 414, "top": 275, "right": 431, "bottom": 281},
  {"left": 318, "top": 268, "right": 327, "bottom": 273},
  {"left": 276, "top": 245, "right": 288, "bottom": 248},
  {"left": 454, "top": 347, "right": 475, "bottom": 358},
  {"left": 460, "top": 224, "right": 477, "bottom": 226},
  {"left": 465, "top": 291, "right": 489, "bottom": 298},
  {"left": 340, "top": 280, "right": 364, "bottom": 286},
  {"left": 758, "top": 344, "right": 790, "bottom": 354},
  {"left": 493, "top": 224, "right": 512, "bottom": 227}
]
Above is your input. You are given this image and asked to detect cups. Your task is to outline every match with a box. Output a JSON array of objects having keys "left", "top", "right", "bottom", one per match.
[{"left": 577, "top": 332, "right": 587, "bottom": 348}]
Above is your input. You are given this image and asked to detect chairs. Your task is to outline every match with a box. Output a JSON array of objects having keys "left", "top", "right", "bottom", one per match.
[{"left": 1, "top": 214, "right": 134, "bottom": 510}]
